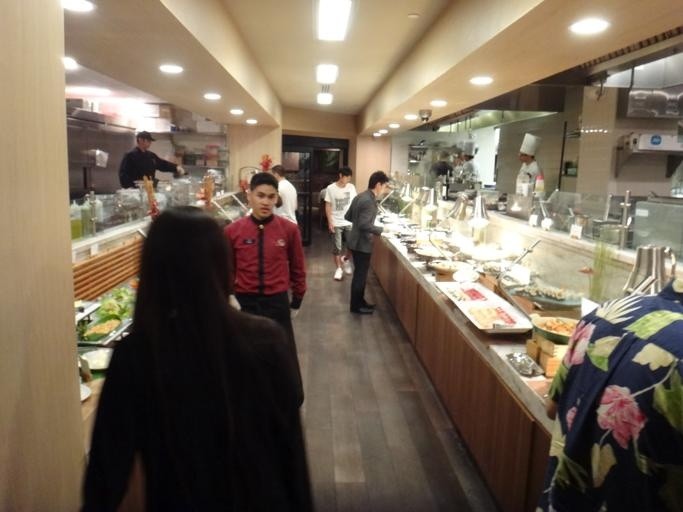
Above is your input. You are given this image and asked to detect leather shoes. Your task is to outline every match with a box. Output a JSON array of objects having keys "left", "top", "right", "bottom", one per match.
[
  {"left": 361, "top": 301, "right": 376, "bottom": 309},
  {"left": 350, "top": 307, "right": 373, "bottom": 314}
]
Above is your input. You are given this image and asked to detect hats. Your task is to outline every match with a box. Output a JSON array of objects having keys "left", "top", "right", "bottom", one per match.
[
  {"left": 137, "top": 131, "right": 155, "bottom": 142},
  {"left": 519, "top": 132, "right": 542, "bottom": 157},
  {"left": 464, "top": 140, "right": 476, "bottom": 156}
]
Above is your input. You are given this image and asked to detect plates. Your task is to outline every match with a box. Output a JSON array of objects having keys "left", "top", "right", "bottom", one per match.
[
  {"left": 531, "top": 317, "right": 580, "bottom": 345},
  {"left": 508, "top": 287, "right": 583, "bottom": 305},
  {"left": 78, "top": 348, "right": 114, "bottom": 369},
  {"left": 453, "top": 269, "right": 480, "bottom": 282},
  {"left": 80, "top": 384, "right": 92, "bottom": 401}
]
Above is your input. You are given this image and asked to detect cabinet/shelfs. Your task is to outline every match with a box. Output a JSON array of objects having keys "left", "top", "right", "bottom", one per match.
[{"left": 403, "top": 171, "right": 683, "bottom": 275}]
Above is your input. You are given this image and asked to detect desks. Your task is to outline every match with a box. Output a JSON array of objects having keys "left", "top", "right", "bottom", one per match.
[
  {"left": 77, "top": 274, "right": 138, "bottom": 453},
  {"left": 370, "top": 213, "right": 488, "bottom": 350},
  {"left": 415, "top": 274, "right": 610, "bottom": 510}
]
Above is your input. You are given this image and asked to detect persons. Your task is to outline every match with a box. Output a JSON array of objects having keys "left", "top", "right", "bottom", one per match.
[
  {"left": 460, "top": 141, "right": 478, "bottom": 183},
  {"left": 222, "top": 172, "right": 307, "bottom": 408},
  {"left": 271, "top": 165, "right": 298, "bottom": 225},
  {"left": 84, "top": 206, "right": 315, "bottom": 511},
  {"left": 428, "top": 151, "right": 452, "bottom": 190},
  {"left": 344, "top": 171, "right": 389, "bottom": 314},
  {"left": 120, "top": 131, "right": 185, "bottom": 189},
  {"left": 515, "top": 133, "right": 544, "bottom": 197},
  {"left": 534, "top": 277, "right": 682, "bottom": 512},
  {"left": 324, "top": 167, "right": 357, "bottom": 280}
]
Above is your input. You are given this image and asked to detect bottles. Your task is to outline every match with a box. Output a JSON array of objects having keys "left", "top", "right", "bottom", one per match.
[
  {"left": 442, "top": 168, "right": 449, "bottom": 200},
  {"left": 535, "top": 176, "right": 544, "bottom": 192}
]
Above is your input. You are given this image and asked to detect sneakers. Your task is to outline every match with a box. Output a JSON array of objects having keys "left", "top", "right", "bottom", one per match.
[
  {"left": 340, "top": 255, "right": 353, "bottom": 274},
  {"left": 333, "top": 268, "right": 344, "bottom": 281}
]
